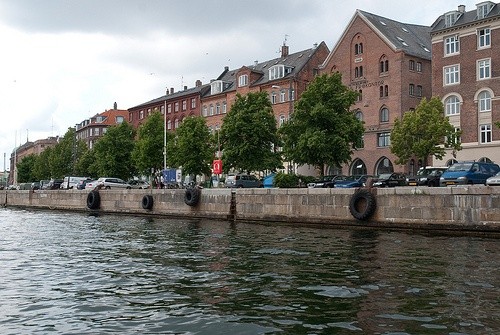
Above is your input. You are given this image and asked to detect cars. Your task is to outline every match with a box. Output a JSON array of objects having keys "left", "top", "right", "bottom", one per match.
[
  {"left": 128, "top": 180, "right": 151, "bottom": 189},
  {"left": 9, "top": 185, "right": 16, "bottom": 190},
  {"left": 404, "top": 168, "right": 449, "bottom": 187},
  {"left": 306, "top": 175, "right": 348, "bottom": 188},
  {"left": 85, "top": 177, "right": 132, "bottom": 191},
  {"left": 485, "top": 172, "right": 500, "bottom": 186},
  {"left": 334, "top": 175, "right": 374, "bottom": 188}
]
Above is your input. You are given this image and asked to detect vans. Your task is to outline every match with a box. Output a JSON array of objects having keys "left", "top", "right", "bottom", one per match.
[
  {"left": 60, "top": 176, "right": 93, "bottom": 190},
  {"left": 39, "top": 179, "right": 50, "bottom": 190},
  {"left": 439, "top": 162, "right": 500, "bottom": 187}
]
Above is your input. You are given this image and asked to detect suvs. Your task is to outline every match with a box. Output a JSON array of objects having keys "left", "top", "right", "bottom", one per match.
[
  {"left": 362, "top": 173, "right": 407, "bottom": 188},
  {"left": 47, "top": 178, "right": 64, "bottom": 190},
  {"left": 77, "top": 178, "right": 98, "bottom": 190},
  {"left": 224, "top": 174, "right": 262, "bottom": 188},
  {"left": 31, "top": 182, "right": 40, "bottom": 190}
]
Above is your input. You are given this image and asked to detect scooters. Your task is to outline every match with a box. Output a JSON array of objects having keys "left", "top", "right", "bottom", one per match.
[{"left": 93, "top": 184, "right": 111, "bottom": 190}]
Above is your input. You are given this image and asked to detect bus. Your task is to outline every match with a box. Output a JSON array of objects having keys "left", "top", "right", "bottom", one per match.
[{"left": 417, "top": 166, "right": 450, "bottom": 176}]
